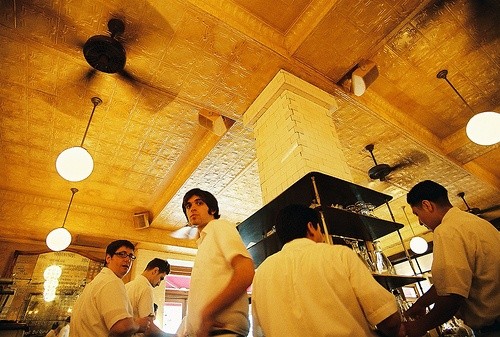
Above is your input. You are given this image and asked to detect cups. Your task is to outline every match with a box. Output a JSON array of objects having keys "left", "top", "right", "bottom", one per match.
[{"left": 344, "top": 201, "right": 376, "bottom": 218}]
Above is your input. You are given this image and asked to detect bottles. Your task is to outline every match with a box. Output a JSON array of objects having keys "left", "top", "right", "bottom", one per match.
[
  {"left": 351, "top": 240, "right": 397, "bottom": 275},
  {"left": 390, "top": 288, "right": 415, "bottom": 322},
  {"left": 439, "top": 315, "right": 475, "bottom": 337}
]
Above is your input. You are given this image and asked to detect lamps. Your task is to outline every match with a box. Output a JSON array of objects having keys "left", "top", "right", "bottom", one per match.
[
  {"left": 436, "top": 70, "right": 500, "bottom": 145},
  {"left": 45, "top": 188, "right": 79, "bottom": 251},
  {"left": 56, "top": 97, "right": 103, "bottom": 181},
  {"left": 399, "top": 206, "right": 429, "bottom": 255}
]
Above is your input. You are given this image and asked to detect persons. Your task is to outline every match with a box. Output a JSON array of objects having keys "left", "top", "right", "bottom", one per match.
[
  {"left": 69, "top": 240, "right": 153, "bottom": 337},
  {"left": 403, "top": 180, "right": 500, "bottom": 337},
  {"left": 125, "top": 258, "right": 180, "bottom": 337},
  {"left": 250, "top": 205, "right": 403, "bottom": 337},
  {"left": 45, "top": 316, "right": 71, "bottom": 337},
  {"left": 174, "top": 188, "right": 255, "bottom": 337}
]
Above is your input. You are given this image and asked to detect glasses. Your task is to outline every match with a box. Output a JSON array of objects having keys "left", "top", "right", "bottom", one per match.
[{"left": 113, "top": 251, "right": 136, "bottom": 261}]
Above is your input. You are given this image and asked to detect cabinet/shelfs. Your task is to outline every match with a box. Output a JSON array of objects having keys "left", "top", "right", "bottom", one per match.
[{"left": 236, "top": 171, "right": 426, "bottom": 337}]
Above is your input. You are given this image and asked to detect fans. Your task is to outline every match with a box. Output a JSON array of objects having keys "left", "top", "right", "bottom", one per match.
[
  {"left": 457, "top": 192, "right": 500, "bottom": 218},
  {"left": 12, "top": 0, "right": 185, "bottom": 120},
  {"left": 347, "top": 143, "right": 430, "bottom": 194}
]
